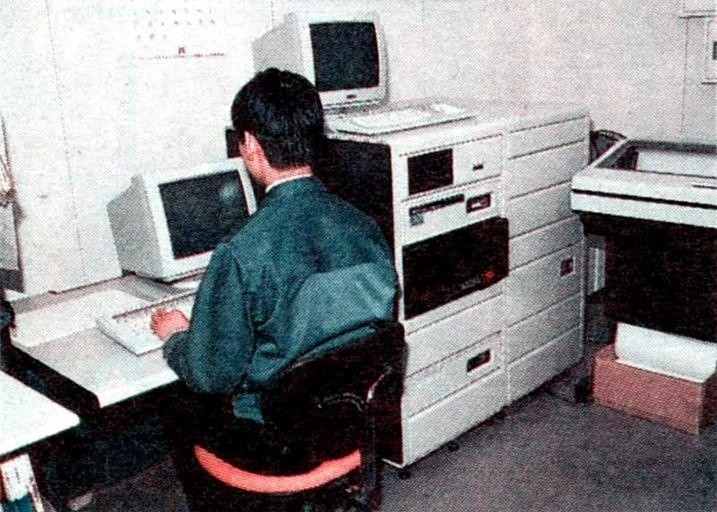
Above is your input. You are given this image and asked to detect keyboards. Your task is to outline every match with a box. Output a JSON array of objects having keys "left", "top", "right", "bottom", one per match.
[
  {"left": 327, "top": 102, "right": 477, "bottom": 136},
  {"left": 96, "top": 289, "right": 199, "bottom": 357}
]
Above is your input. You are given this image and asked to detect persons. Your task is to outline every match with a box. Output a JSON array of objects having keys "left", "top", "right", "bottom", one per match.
[{"left": 149, "top": 66, "right": 402, "bottom": 512}]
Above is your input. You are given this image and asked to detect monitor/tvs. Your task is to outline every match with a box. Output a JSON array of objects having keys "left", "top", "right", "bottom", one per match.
[
  {"left": 106, "top": 156, "right": 258, "bottom": 289},
  {"left": 253, "top": 13, "right": 387, "bottom": 113}
]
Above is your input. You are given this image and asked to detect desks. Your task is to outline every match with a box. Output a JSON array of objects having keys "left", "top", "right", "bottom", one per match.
[{"left": 1, "top": 275, "right": 399, "bottom": 512}]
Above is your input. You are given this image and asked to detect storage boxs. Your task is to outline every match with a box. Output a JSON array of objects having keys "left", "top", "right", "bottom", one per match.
[{"left": 594, "top": 323, "right": 717, "bottom": 434}]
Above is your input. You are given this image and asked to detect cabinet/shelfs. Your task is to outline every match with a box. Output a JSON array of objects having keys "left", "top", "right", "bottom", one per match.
[{"left": 225, "top": 98, "right": 590, "bottom": 478}]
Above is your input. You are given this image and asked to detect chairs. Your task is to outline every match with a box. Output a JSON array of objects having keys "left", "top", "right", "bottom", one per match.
[{"left": 192, "top": 322, "right": 407, "bottom": 512}]
